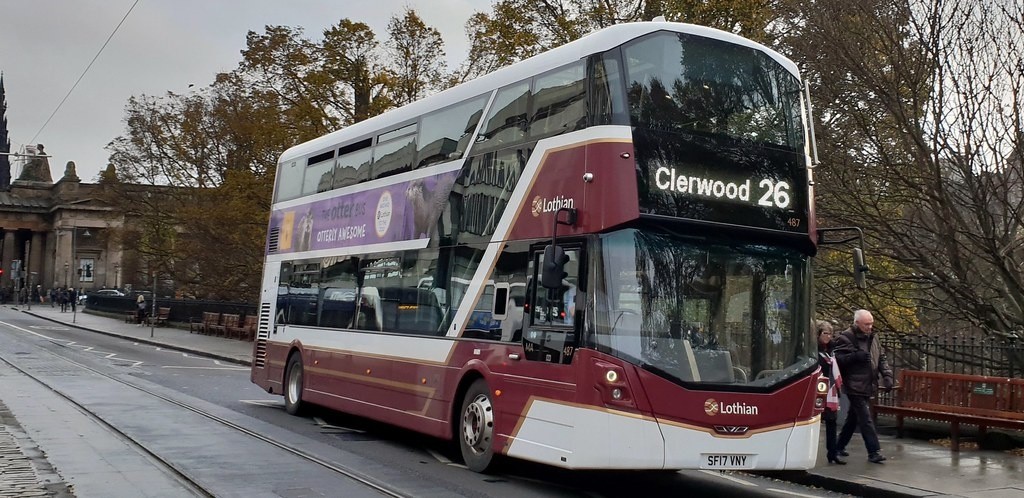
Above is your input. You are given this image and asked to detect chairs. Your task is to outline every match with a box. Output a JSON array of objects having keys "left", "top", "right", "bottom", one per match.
[{"left": 277, "top": 276, "right": 517, "bottom": 340}]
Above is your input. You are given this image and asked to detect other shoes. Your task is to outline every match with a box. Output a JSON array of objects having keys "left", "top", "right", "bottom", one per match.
[
  {"left": 837, "top": 448, "right": 849, "bottom": 457},
  {"left": 868, "top": 450, "right": 886, "bottom": 462}
]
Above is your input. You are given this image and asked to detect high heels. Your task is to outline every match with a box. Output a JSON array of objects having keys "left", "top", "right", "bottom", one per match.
[{"left": 826, "top": 452, "right": 848, "bottom": 465}]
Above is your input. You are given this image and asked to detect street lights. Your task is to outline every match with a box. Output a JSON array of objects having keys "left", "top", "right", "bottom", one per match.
[{"left": 62, "top": 262, "right": 70, "bottom": 313}]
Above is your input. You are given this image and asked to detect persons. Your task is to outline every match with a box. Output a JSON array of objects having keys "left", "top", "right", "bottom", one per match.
[
  {"left": 19, "top": 280, "right": 106, "bottom": 314},
  {"left": 833, "top": 309, "right": 894, "bottom": 463},
  {"left": 135, "top": 294, "right": 148, "bottom": 328},
  {"left": 814, "top": 319, "right": 848, "bottom": 466},
  {"left": 15, "top": 144, "right": 53, "bottom": 182}
]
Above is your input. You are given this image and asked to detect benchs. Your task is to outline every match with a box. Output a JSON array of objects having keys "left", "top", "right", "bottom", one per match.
[
  {"left": 228, "top": 315, "right": 258, "bottom": 343},
  {"left": 145, "top": 307, "right": 171, "bottom": 327},
  {"left": 868, "top": 369, "right": 1024, "bottom": 455},
  {"left": 208, "top": 314, "right": 240, "bottom": 338},
  {"left": 190, "top": 312, "right": 220, "bottom": 335}
]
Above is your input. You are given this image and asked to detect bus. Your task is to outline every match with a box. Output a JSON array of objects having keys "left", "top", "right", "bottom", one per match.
[{"left": 242, "top": 19, "right": 869, "bottom": 478}]
